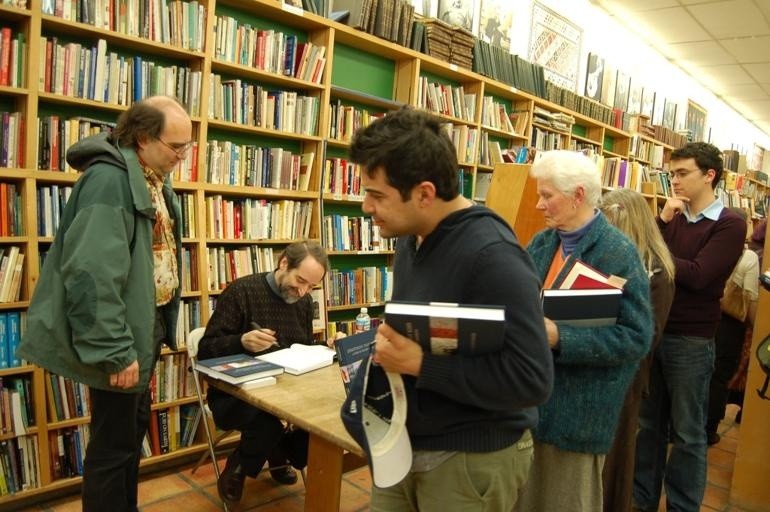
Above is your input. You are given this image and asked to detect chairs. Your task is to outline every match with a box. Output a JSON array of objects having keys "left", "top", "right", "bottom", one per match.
[{"left": 187, "top": 328, "right": 307, "bottom": 512}]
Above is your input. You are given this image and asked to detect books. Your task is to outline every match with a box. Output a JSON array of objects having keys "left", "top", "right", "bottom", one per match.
[
  {"left": 0, "top": 0, "right": 42, "bottom": 494},
  {"left": 206, "top": 0, "right": 327, "bottom": 329},
  {"left": 340, "top": 0, "right": 769, "bottom": 219},
  {"left": 37, "top": 1, "right": 204, "bottom": 481},
  {"left": 327, "top": 103, "right": 401, "bottom": 340}
]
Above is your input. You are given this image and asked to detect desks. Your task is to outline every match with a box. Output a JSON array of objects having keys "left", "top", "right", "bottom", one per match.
[{"left": 204, "top": 347, "right": 368, "bottom": 512}]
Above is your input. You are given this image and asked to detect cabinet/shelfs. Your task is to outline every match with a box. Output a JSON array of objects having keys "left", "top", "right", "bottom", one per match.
[{"left": 0, "top": 0, "right": 770, "bottom": 511}]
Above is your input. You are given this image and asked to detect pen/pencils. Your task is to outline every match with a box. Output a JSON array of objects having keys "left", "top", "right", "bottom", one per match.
[{"left": 250, "top": 321, "right": 281, "bottom": 348}]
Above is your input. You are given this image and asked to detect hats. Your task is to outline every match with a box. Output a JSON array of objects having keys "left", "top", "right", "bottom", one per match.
[
  {"left": 340, "top": 347, "right": 414, "bottom": 488},
  {"left": 750, "top": 220, "right": 767, "bottom": 240}
]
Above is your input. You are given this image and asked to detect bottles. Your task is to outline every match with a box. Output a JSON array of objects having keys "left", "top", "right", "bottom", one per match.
[{"left": 355, "top": 307, "right": 372, "bottom": 337}]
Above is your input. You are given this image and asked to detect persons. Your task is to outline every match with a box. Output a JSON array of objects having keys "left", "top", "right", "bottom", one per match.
[
  {"left": 703, "top": 206, "right": 760, "bottom": 443},
  {"left": 517, "top": 146, "right": 653, "bottom": 511},
  {"left": 349, "top": 106, "right": 555, "bottom": 510},
  {"left": 630, "top": 141, "right": 747, "bottom": 510},
  {"left": 198, "top": 240, "right": 331, "bottom": 506},
  {"left": 16, "top": 96, "right": 192, "bottom": 512},
  {"left": 597, "top": 184, "right": 676, "bottom": 510}
]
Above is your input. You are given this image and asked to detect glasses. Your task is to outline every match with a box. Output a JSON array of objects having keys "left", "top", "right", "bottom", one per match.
[
  {"left": 667, "top": 168, "right": 701, "bottom": 181},
  {"left": 156, "top": 136, "right": 192, "bottom": 155}
]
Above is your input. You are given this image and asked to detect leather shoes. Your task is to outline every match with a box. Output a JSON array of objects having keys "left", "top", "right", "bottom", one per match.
[
  {"left": 217, "top": 445, "right": 246, "bottom": 505},
  {"left": 268, "top": 450, "right": 298, "bottom": 484},
  {"left": 707, "top": 433, "right": 720, "bottom": 446}
]
